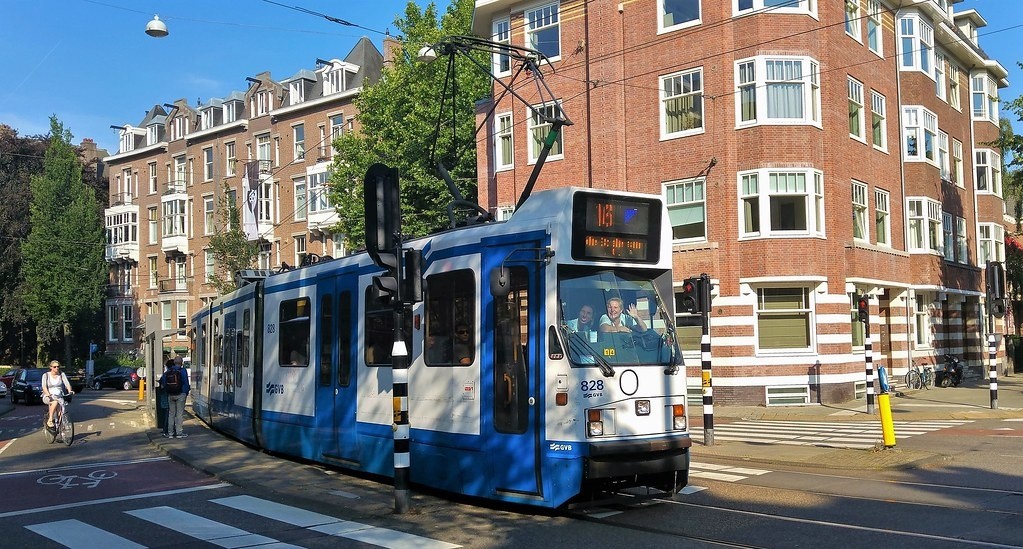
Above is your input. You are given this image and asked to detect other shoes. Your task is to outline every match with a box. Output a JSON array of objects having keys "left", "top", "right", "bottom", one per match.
[
  {"left": 47, "top": 420, "right": 54, "bottom": 427},
  {"left": 162, "top": 433, "right": 168, "bottom": 437}
]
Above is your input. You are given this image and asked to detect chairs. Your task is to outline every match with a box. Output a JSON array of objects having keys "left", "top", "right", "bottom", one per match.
[
  {"left": 367, "top": 343, "right": 387, "bottom": 364},
  {"left": 428, "top": 336, "right": 448, "bottom": 362}
]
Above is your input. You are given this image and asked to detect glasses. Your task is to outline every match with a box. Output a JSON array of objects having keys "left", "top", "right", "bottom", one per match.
[
  {"left": 458, "top": 330, "right": 470, "bottom": 334},
  {"left": 51, "top": 365, "right": 60, "bottom": 368}
]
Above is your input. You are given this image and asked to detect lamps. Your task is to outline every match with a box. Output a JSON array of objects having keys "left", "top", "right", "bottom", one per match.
[
  {"left": 144, "top": 14, "right": 169, "bottom": 37},
  {"left": 418, "top": 43, "right": 437, "bottom": 64}
]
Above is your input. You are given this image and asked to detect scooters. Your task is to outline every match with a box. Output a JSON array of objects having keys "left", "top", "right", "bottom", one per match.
[{"left": 941, "top": 352, "right": 966, "bottom": 389}]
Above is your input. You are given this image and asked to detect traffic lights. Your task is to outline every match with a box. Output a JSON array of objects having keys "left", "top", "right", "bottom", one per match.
[
  {"left": 682, "top": 278, "right": 699, "bottom": 314},
  {"left": 857, "top": 297, "right": 870, "bottom": 322},
  {"left": 706, "top": 276, "right": 718, "bottom": 314}
]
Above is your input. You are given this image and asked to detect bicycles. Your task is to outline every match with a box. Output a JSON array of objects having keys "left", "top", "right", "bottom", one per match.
[
  {"left": 904, "top": 357, "right": 935, "bottom": 391},
  {"left": 43, "top": 391, "right": 75, "bottom": 446}
]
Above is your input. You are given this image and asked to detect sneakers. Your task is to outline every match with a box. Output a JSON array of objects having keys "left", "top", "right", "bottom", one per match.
[
  {"left": 176, "top": 433, "right": 188, "bottom": 438},
  {"left": 169, "top": 433, "right": 174, "bottom": 438}
]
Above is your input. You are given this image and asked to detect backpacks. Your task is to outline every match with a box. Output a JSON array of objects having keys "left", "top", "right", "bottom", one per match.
[{"left": 166, "top": 366, "right": 183, "bottom": 395}]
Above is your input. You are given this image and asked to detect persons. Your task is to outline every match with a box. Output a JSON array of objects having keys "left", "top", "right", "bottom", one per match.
[
  {"left": 41, "top": 360, "right": 76, "bottom": 429},
  {"left": 599, "top": 298, "right": 647, "bottom": 333},
  {"left": 158, "top": 356, "right": 191, "bottom": 439},
  {"left": 565, "top": 301, "right": 597, "bottom": 332},
  {"left": 427, "top": 322, "right": 473, "bottom": 364}
]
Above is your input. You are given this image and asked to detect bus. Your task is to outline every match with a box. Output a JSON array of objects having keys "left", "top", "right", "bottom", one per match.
[{"left": 188, "top": 35, "right": 694, "bottom": 516}]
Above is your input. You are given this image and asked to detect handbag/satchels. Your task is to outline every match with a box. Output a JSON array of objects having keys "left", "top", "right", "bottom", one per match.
[{"left": 40, "top": 392, "right": 50, "bottom": 404}]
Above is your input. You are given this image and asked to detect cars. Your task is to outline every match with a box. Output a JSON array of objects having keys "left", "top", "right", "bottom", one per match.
[
  {"left": 10, "top": 367, "right": 72, "bottom": 406},
  {"left": 0, "top": 368, "right": 22, "bottom": 388},
  {"left": 0, "top": 379, "right": 8, "bottom": 397},
  {"left": 92, "top": 365, "right": 147, "bottom": 390},
  {"left": 65, "top": 372, "right": 85, "bottom": 393}
]
